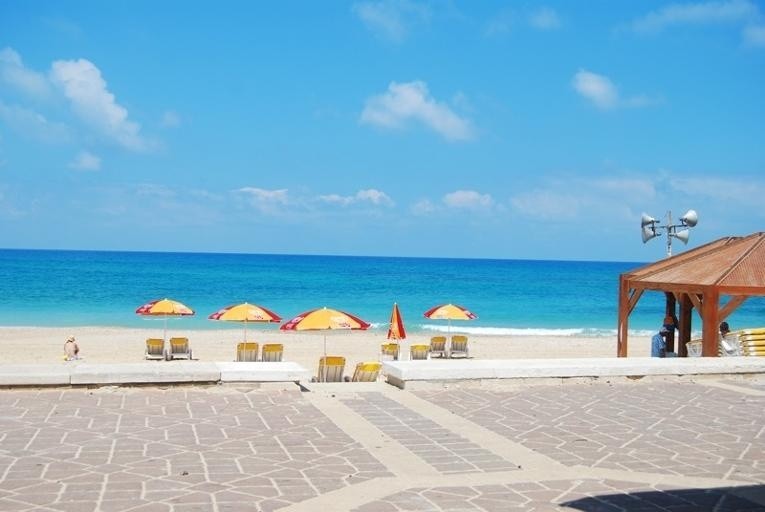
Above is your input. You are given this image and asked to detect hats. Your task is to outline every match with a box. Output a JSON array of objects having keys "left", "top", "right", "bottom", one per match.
[
  {"left": 660, "top": 327, "right": 670, "bottom": 333},
  {"left": 66, "top": 335, "right": 75, "bottom": 342}
]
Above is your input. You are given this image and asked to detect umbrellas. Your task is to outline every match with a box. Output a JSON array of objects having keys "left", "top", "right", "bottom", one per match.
[
  {"left": 136, "top": 297, "right": 195, "bottom": 361},
  {"left": 387, "top": 302, "right": 406, "bottom": 361},
  {"left": 423, "top": 302, "right": 480, "bottom": 359},
  {"left": 279, "top": 306, "right": 372, "bottom": 356},
  {"left": 208, "top": 301, "right": 282, "bottom": 362}
]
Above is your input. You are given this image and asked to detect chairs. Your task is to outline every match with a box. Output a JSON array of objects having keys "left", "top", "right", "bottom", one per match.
[
  {"left": 309, "top": 354, "right": 385, "bottom": 383},
  {"left": 380, "top": 332, "right": 472, "bottom": 362},
  {"left": 143, "top": 337, "right": 193, "bottom": 362},
  {"left": 233, "top": 341, "right": 286, "bottom": 363}
]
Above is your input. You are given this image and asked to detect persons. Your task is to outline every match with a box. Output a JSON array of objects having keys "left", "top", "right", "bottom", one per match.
[
  {"left": 719, "top": 322, "right": 739, "bottom": 357},
  {"left": 64, "top": 335, "right": 80, "bottom": 360},
  {"left": 651, "top": 327, "right": 670, "bottom": 358}
]
